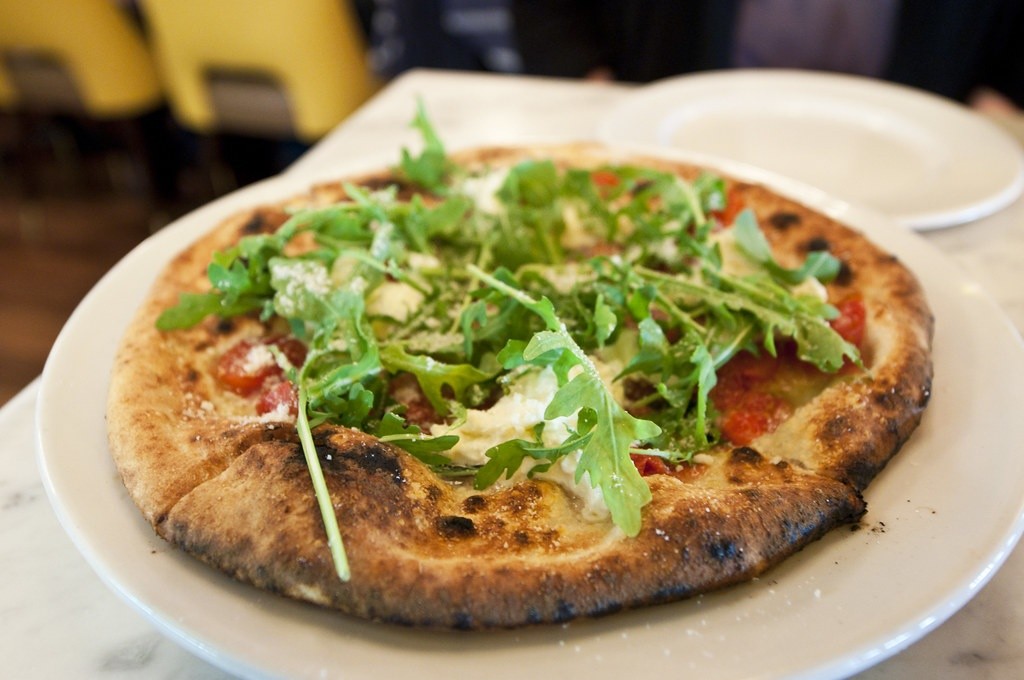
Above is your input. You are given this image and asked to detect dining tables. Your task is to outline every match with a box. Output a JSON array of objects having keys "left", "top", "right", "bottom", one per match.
[{"left": 0, "top": 67, "right": 1024, "bottom": 680}]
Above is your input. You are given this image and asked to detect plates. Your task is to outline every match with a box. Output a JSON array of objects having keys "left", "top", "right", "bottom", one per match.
[
  {"left": 607, "top": 63, "right": 1021, "bottom": 235},
  {"left": 34, "top": 146, "right": 1023, "bottom": 680}
]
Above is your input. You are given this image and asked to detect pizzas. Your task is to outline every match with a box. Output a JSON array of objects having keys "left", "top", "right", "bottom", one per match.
[{"left": 103, "top": 101, "right": 936, "bottom": 627}]
